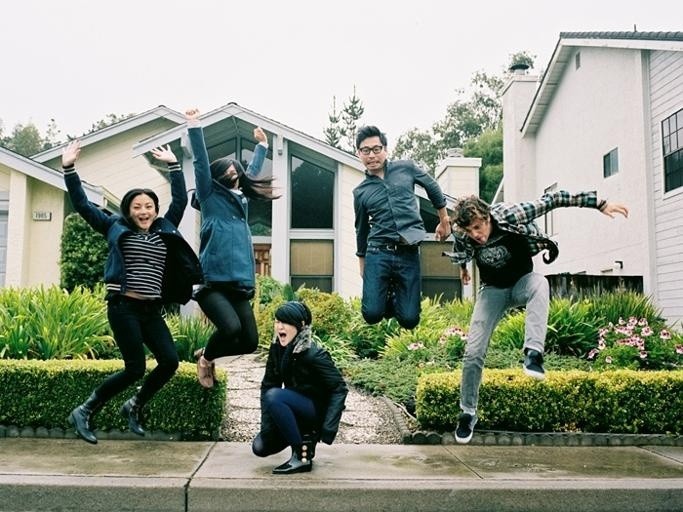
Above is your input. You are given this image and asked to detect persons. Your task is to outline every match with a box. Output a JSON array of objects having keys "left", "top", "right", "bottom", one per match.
[
  {"left": 250, "top": 301, "right": 348, "bottom": 475},
  {"left": 437, "top": 188, "right": 633, "bottom": 443},
  {"left": 184, "top": 106, "right": 282, "bottom": 386},
  {"left": 351, "top": 125, "right": 452, "bottom": 329},
  {"left": 58, "top": 138, "right": 207, "bottom": 445}
]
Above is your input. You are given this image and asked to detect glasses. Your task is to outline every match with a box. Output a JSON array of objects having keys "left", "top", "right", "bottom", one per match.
[{"left": 359, "top": 146, "right": 384, "bottom": 155}]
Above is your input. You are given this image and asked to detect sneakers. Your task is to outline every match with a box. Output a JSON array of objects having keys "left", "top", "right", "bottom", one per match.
[
  {"left": 193, "top": 348, "right": 215, "bottom": 388},
  {"left": 455, "top": 414, "right": 479, "bottom": 443},
  {"left": 522, "top": 347, "right": 545, "bottom": 378}
]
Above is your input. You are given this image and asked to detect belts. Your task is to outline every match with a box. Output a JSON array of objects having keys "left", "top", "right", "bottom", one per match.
[{"left": 379, "top": 244, "right": 413, "bottom": 251}]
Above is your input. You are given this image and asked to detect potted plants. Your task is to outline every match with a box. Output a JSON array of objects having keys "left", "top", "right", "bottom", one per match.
[
  {"left": 446, "top": 140, "right": 462, "bottom": 157},
  {"left": 508, "top": 48, "right": 536, "bottom": 74}
]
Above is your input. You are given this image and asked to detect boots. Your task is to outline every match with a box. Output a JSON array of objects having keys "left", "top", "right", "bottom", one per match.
[
  {"left": 273, "top": 431, "right": 317, "bottom": 474},
  {"left": 120, "top": 394, "right": 145, "bottom": 436},
  {"left": 67, "top": 390, "right": 102, "bottom": 443}
]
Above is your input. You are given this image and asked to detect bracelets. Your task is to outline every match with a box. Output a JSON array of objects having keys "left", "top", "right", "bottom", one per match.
[{"left": 597, "top": 198, "right": 607, "bottom": 212}]
